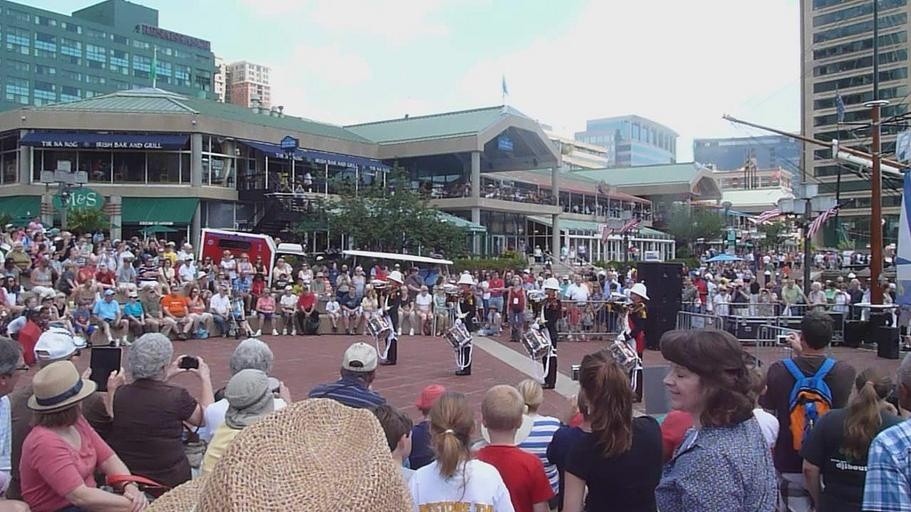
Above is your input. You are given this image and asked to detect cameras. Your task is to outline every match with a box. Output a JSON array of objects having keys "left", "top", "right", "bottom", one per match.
[
  {"left": 776, "top": 335, "right": 790, "bottom": 345},
  {"left": 179, "top": 355, "right": 197, "bottom": 370}
]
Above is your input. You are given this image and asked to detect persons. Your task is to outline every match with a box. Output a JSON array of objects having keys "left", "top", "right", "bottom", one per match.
[
  {"left": 366, "top": 271, "right": 404, "bottom": 364},
  {"left": 862, "top": 353, "right": 911, "bottom": 511},
  {"left": 0, "top": 215, "right": 368, "bottom": 346},
  {"left": 656, "top": 408, "right": 694, "bottom": 474},
  {"left": 526, "top": 279, "right": 564, "bottom": 390},
  {"left": 802, "top": 364, "right": 899, "bottom": 511},
  {"left": 758, "top": 312, "right": 859, "bottom": 512},
  {"left": 472, "top": 292, "right": 526, "bottom": 342},
  {"left": 467, "top": 412, "right": 535, "bottom": 453},
  {"left": 269, "top": 172, "right": 557, "bottom": 205},
  {"left": 564, "top": 347, "right": 664, "bottom": 512},
  {"left": 200, "top": 368, "right": 291, "bottom": 473},
  {"left": 409, "top": 391, "right": 517, "bottom": 512},
  {"left": 397, "top": 285, "right": 456, "bottom": 339},
  {"left": 411, "top": 383, "right": 446, "bottom": 470},
  {"left": 373, "top": 404, "right": 417, "bottom": 482},
  {"left": 203, "top": 338, "right": 294, "bottom": 448},
  {"left": 10, "top": 327, "right": 129, "bottom": 511},
  {"left": 732, "top": 353, "right": 781, "bottom": 468},
  {"left": 859, "top": 242, "right": 911, "bottom": 352},
  {"left": 113, "top": 332, "right": 216, "bottom": 503},
  {"left": 681, "top": 239, "right": 858, "bottom": 326},
  {"left": 560, "top": 199, "right": 650, "bottom": 219},
  {"left": 660, "top": 327, "right": 778, "bottom": 510},
  {"left": 92, "top": 159, "right": 106, "bottom": 181},
  {"left": 546, "top": 387, "right": 592, "bottom": 512},
  {"left": 307, "top": 341, "right": 388, "bottom": 412},
  {"left": 18, "top": 359, "right": 152, "bottom": 511},
  {"left": 473, "top": 384, "right": 557, "bottom": 512},
  {"left": 445, "top": 274, "right": 479, "bottom": 376},
  {"left": 188, "top": 385, "right": 227, "bottom": 480},
  {"left": 516, "top": 378, "right": 565, "bottom": 511},
  {"left": 611, "top": 282, "right": 650, "bottom": 404},
  {"left": 367, "top": 239, "right": 639, "bottom": 291},
  {"left": 560, "top": 291, "right": 626, "bottom": 341},
  {"left": 0, "top": 336, "right": 31, "bottom": 512},
  {"left": 897, "top": 132, "right": 911, "bottom": 156},
  {"left": 877, "top": 383, "right": 911, "bottom": 420}
]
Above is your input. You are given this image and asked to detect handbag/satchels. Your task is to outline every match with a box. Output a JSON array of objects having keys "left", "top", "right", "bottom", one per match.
[{"left": 198, "top": 327, "right": 208, "bottom": 339}]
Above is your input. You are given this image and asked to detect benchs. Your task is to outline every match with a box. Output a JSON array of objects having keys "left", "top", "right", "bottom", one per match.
[
  {"left": 18, "top": 272, "right": 225, "bottom": 346},
  {"left": 246, "top": 294, "right": 432, "bottom": 336}
]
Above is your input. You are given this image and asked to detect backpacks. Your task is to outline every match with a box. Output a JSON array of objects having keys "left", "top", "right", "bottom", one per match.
[{"left": 782, "top": 358, "right": 838, "bottom": 457}]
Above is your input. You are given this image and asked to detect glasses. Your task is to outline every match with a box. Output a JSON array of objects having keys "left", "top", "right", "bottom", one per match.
[{"left": 15, "top": 367, "right": 29, "bottom": 373}]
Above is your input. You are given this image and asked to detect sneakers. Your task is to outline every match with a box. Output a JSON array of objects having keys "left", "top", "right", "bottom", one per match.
[
  {"left": 272, "top": 328, "right": 279, "bottom": 335},
  {"left": 410, "top": 328, "right": 414, "bottom": 335},
  {"left": 282, "top": 329, "right": 287, "bottom": 336},
  {"left": 398, "top": 328, "right": 402, "bottom": 336},
  {"left": 253, "top": 329, "right": 262, "bottom": 336},
  {"left": 291, "top": 330, "right": 296, "bottom": 335},
  {"left": 178, "top": 332, "right": 190, "bottom": 340}
]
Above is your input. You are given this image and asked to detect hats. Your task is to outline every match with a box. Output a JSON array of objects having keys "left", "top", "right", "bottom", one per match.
[
  {"left": 458, "top": 274, "right": 477, "bottom": 285},
  {"left": 661, "top": 329, "right": 745, "bottom": 373},
  {"left": 104, "top": 290, "right": 115, "bottom": 295},
  {"left": 34, "top": 327, "right": 86, "bottom": 361},
  {"left": 185, "top": 256, "right": 192, "bottom": 261},
  {"left": 197, "top": 272, "right": 208, "bottom": 280},
  {"left": 342, "top": 342, "right": 378, "bottom": 373},
  {"left": 286, "top": 286, "right": 293, "bottom": 290},
  {"left": 128, "top": 292, "right": 138, "bottom": 297},
  {"left": 27, "top": 361, "right": 97, "bottom": 414},
  {"left": 543, "top": 278, "right": 562, "bottom": 291},
  {"left": 626, "top": 284, "right": 650, "bottom": 301},
  {"left": 224, "top": 369, "right": 278, "bottom": 429},
  {"left": 386, "top": 271, "right": 404, "bottom": 285},
  {"left": 416, "top": 385, "right": 446, "bottom": 410}
]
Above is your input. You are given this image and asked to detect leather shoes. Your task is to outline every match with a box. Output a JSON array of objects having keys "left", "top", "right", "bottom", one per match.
[
  {"left": 540, "top": 384, "right": 555, "bottom": 389},
  {"left": 379, "top": 359, "right": 396, "bottom": 365},
  {"left": 455, "top": 369, "right": 470, "bottom": 375}
]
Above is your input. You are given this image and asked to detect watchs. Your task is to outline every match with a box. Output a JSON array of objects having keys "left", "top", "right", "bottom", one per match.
[{"left": 122, "top": 480, "right": 139, "bottom": 495}]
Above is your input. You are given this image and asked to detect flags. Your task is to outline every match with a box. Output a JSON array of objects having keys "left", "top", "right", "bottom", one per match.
[
  {"left": 748, "top": 208, "right": 783, "bottom": 225},
  {"left": 806, "top": 206, "right": 840, "bottom": 240},
  {"left": 836, "top": 91, "right": 846, "bottom": 123},
  {"left": 503, "top": 76, "right": 508, "bottom": 95},
  {"left": 602, "top": 218, "right": 640, "bottom": 244},
  {"left": 149, "top": 47, "right": 157, "bottom": 82}
]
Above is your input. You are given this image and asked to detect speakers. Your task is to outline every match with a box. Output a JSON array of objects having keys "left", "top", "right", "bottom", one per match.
[
  {"left": 635, "top": 262, "right": 683, "bottom": 351},
  {"left": 877, "top": 326, "right": 900, "bottom": 359}
]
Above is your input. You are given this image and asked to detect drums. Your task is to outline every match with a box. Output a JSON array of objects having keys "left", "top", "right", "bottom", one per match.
[
  {"left": 608, "top": 339, "right": 640, "bottom": 372},
  {"left": 444, "top": 319, "right": 472, "bottom": 351},
  {"left": 367, "top": 312, "right": 391, "bottom": 340},
  {"left": 520, "top": 328, "right": 552, "bottom": 360}
]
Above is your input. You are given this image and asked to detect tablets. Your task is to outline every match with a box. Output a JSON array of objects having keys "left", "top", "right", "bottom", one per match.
[{"left": 89, "top": 346, "right": 122, "bottom": 392}]
